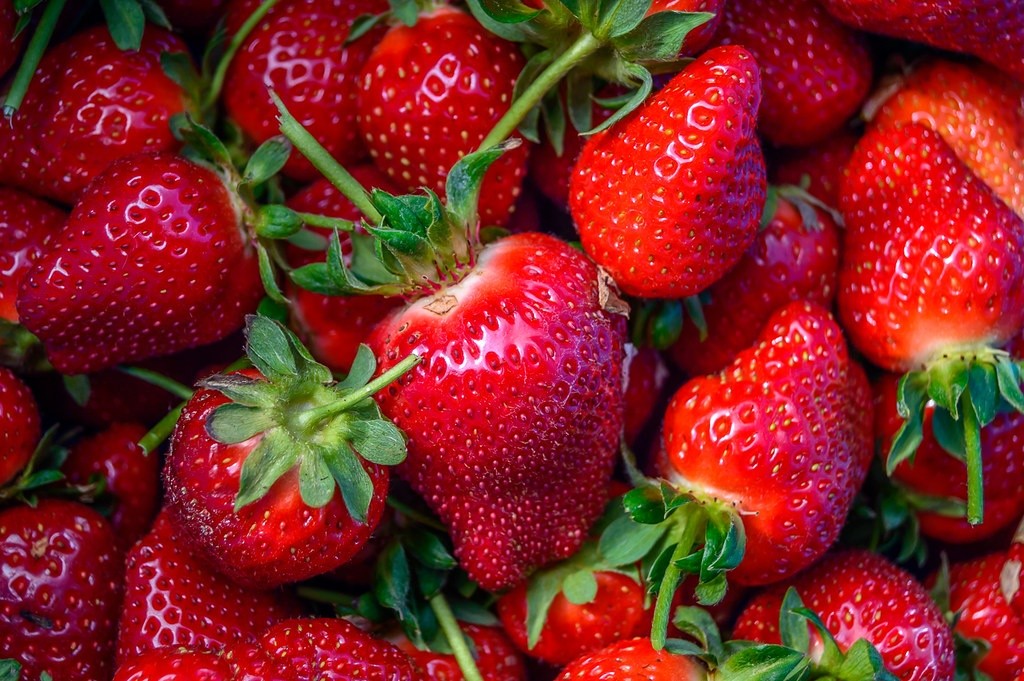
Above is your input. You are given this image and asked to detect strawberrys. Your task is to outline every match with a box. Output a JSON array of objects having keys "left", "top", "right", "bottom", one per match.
[{"left": 0, "top": 0, "right": 1024, "bottom": 681}]
